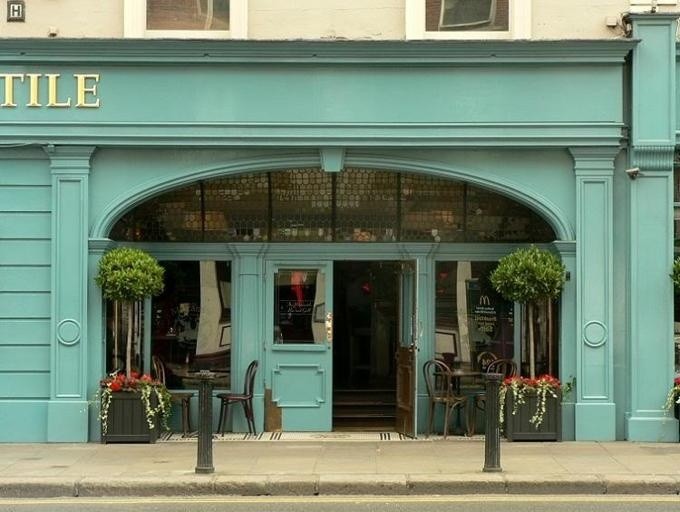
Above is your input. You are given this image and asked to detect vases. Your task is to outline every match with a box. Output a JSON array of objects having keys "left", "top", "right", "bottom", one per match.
[
  {"left": 101, "top": 388, "right": 162, "bottom": 443},
  {"left": 503, "top": 386, "right": 562, "bottom": 441}
]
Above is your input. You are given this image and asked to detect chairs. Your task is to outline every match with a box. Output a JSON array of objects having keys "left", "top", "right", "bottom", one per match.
[
  {"left": 422, "top": 350, "right": 518, "bottom": 440},
  {"left": 151, "top": 354, "right": 259, "bottom": 437}
]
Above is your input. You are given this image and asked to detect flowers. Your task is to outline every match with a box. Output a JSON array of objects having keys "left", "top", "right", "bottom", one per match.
[
  {"left": 89, "top": 366, "right": 178, "bottom": 436},
  {"left": 497, "top": 368, "right": 567, "bottom": 430}
]
